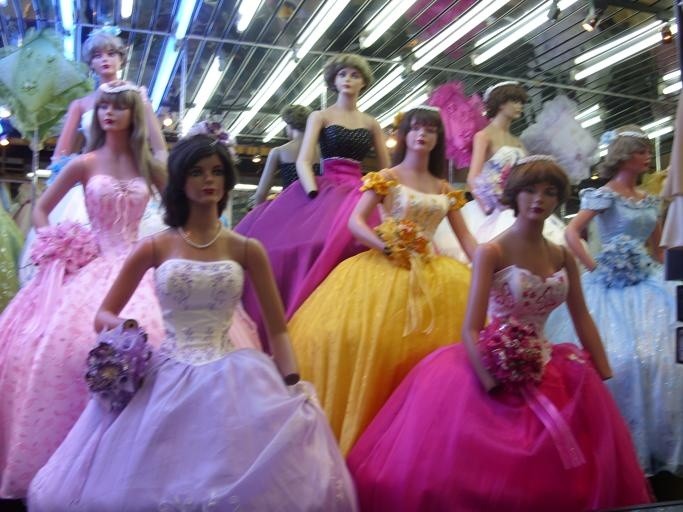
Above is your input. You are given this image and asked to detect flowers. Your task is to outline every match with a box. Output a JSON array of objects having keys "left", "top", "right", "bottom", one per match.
[
  {"left": 28, "top": 218, "right": 97, "bottom": 275},
  {"left": 471, "top": 146, "right": 526, "bottom": 213},
  {"left": 477, "top": 315, "right": 546, "bottom": 388},
  {"left": 81, "top": 323, "right": 152, "bottom": 413},
  {"left": 374, "top": 216, "right": 429, "bottom": 269},
  {"left": 493, "top": 163, "right": 511, "bottom": 199},
  {"left": 46, "top": 152, "right": 79, "bottom": 187},
  {"left": 593, "top": 232, "right": 653, "bottom": 289}
]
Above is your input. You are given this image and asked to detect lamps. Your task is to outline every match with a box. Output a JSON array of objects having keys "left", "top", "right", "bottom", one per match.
[
  {"left": 572, "top": 19, "right": 683, "bottom": 150},
  {"left": 60, "top": 1, "right": 74, "bottom": 62},
  {"left": 114, "top": 0, "right": 134, "bottom": 81},
  {"left": 149, "top": 0, "right": 353, "bottom": 142},
  {"left": 354, "top": 1, "right": 572, "bottom": 149}
]
